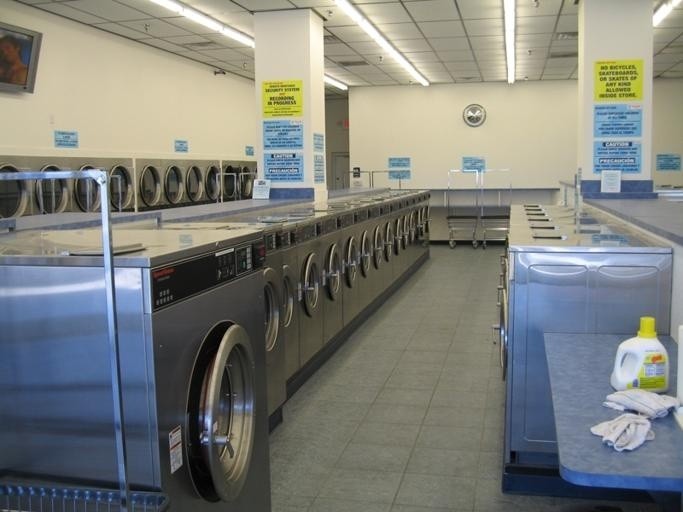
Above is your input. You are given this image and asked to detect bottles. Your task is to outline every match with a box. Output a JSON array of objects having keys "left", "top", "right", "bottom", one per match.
[{"left": 607, "top": 314, "right": 671, "bottom": 395}]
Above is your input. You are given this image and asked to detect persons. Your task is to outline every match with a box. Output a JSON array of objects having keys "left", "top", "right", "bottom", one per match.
[{"left": 1, "top": 35, "right": 28, "bottom": 86}]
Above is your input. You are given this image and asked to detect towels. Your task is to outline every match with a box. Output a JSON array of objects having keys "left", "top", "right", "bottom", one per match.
[
  {"left": 588, "top": 412, "right": 656, "bottom": 454},
  {"left": 597, "top": 382, "right": 680, "bottom": 422}
]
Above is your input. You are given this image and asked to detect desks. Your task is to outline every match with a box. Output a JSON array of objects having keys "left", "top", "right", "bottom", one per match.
[{"left": 542, "top": 325, "right": 682, "bottom": 512}]
